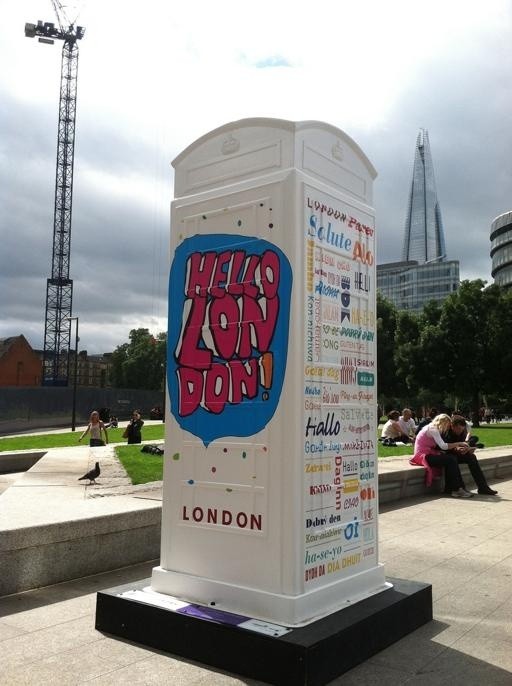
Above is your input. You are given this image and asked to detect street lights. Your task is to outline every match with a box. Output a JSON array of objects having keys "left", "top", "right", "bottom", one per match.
[
  {"left": 160, "top": 361, "right": 167, "bottom": 423},
  {"left": 64, "top": 315, "right": 82, "bottom": 432}
]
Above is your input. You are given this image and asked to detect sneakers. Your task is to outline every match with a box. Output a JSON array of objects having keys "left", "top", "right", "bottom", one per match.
[{"left": 452, "top": 486, "right": 498, "bottom": 497}]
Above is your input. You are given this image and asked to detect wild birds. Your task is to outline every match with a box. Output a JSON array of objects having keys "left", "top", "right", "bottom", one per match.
[{"left": 78, "top": 462, "right": 100, "bottom": 485}]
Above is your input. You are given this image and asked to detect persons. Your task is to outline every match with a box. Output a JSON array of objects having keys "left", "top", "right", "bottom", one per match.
[
  {"left": 122, "top": 408, "right": 144, "bottom": 443},
  {"left": 377, "top": 400, "right": 503, "bottom": 443},
  {"left": 149, "top": 406, "right": 161, "bottom": 419},
  {"left": 77, "top": 409, "right": 110, "bottom": 448},
  {"left": 444, "top": 414, "right": 498, "bottom": 495},
  {"left": 409, "top": 412, "right": 474, "bottom": 497},
  {"left": 414, "top": 405, "right": 466, "bottom": 494},
  {"left": 108, "top": 414, "right": 118, "bottom": 428}
]
